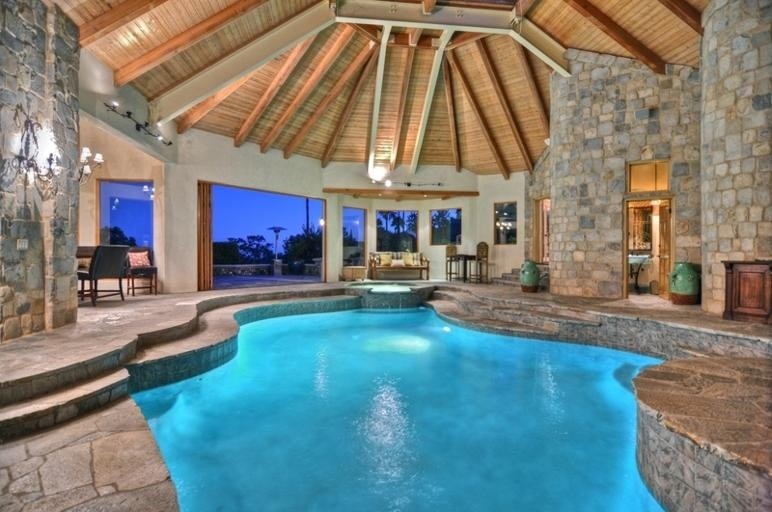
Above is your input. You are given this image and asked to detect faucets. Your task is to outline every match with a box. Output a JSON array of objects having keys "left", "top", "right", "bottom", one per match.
[{"left": 631, "top": 250, "right": 635, "bottom": 255}]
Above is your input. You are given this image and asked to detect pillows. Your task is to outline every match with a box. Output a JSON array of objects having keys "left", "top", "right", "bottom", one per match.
[{"left": 380, "top": 255, "right": 413, "bottom": 268}]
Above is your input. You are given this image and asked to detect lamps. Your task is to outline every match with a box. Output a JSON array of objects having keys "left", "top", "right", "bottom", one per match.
[
  {"left": 142, "top": 184, "right": 154, "bottom": 201},
  {"left": 19, "top": 123, "right": 104, "bottom": 186},
  {"left": 103, "top": 100, "right": 172, "bottom": 147}
]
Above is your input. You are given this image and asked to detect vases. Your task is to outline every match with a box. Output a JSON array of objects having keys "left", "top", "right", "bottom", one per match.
[
  {"left": 670, "top": 262, "right": 701, "bottom": 305},
  {"left": 520, "top": 261, "right": 540, "bottom": 292}
]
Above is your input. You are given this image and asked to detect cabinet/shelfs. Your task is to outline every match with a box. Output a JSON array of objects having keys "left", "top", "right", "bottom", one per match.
[
  {"left": 659, "top": 201, "right": 670, "bottom": 301},
  {"left": 721, "top": 259, "right": 772, "bottom": 325}
]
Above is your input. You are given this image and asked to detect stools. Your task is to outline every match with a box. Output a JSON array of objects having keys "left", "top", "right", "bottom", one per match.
[{"left": 344, "top": 266, "right": 367, "bottom": 282}]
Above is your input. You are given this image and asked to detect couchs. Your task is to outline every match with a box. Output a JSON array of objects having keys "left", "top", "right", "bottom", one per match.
[{"left": 370, "top": 251, "right": 430, "bottom": 280}]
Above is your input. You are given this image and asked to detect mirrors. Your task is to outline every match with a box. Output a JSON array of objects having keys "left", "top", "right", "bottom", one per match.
[{"left": 628, "top": 205, "right": 654, "bottom": 254}]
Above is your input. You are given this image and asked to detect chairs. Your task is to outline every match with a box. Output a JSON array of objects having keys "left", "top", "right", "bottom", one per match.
[
  {"left": 446, "top": 241, "right": 489, "bottom": 284},
  {"left": 76, "top": 245, "right": 158, "bottom": 307}
]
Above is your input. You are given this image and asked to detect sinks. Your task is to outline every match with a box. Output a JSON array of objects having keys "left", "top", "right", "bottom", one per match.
[{"left": 627, "top": 255, "right": 650, "bottom": 264}]
